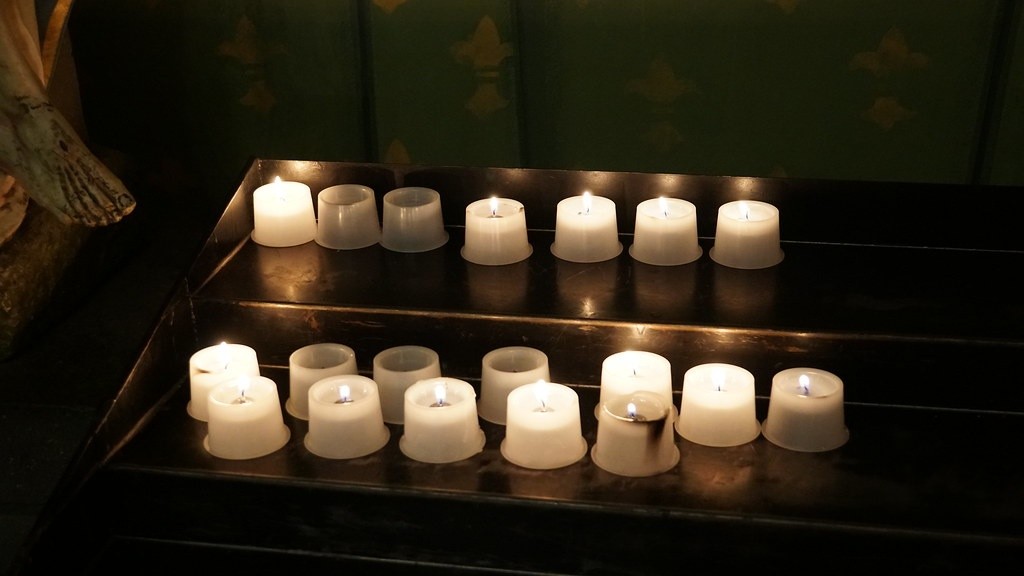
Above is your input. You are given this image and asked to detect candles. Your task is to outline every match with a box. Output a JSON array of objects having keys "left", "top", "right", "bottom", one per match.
[
  {"left": 760, "top": 367, "right": 851, "bottom": 453},
  {"left": 284, "top": 343, "right": 359, "bottom": 422},
  {"left": 459, "top": 196, "right": 536, "bottom": 268},
  {"left": 591, "top": 349, "right": 681, "bottom": 478},
  {"left": 674, "top": 362, "right": 761, "bottom": 448},
  {"left": 628, "top": 193, "right": 706, "bottom": 267},
  {"left": 499, "top": 380, "right": 589, "bottom": 470},
  {"left": 550, "top": 188, "right": 626, "bottom": 265},
  {"left": 304, "top": 374, "right": 392, "bottom": 460},
  {"left": 477, "top": 347, "right": 550, "bottom": 425},
  {"left": 251, "top": 180, "right": 314, "bottom": 249},
  {"left": 399, "top": 375, "right": 487, "bottom": 464},
  {"left": 186, "top": 339, "right": 292, "bottom": 460},
  {"left": 371, "top": 344, "right": 442, "bottom": 424},
  {"left": 316, "top": 183, "right": 377, "bottom": 250},
  {"left": 378, "top": 186, "right": 450, "bottom": 252},
  {"left": 708, "top": 199, "right": 786, "bottom": 271}
]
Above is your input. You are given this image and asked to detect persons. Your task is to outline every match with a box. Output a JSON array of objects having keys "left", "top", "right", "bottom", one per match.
[{"left": 0, "top": 0, "right": 136, "bottom": 226}]
